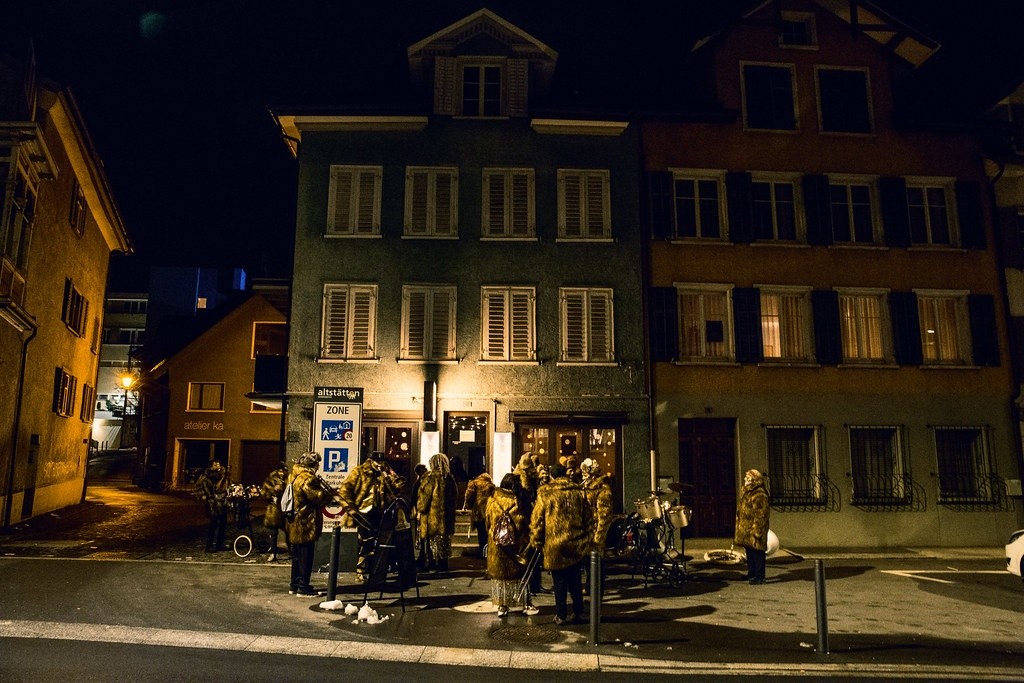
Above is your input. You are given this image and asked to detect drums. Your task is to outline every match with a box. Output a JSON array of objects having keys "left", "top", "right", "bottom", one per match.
[
  {"left": 633, "top": 496, "right": 663, "bottom": 531},
  {"left": 667, "top": 505, "right": 689, "bottom": 529},
  {"left": 660, "top": 500, "right": 672, "bottom": 512}
]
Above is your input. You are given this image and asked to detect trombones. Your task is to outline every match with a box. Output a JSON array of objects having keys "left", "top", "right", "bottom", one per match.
[
  {"left": 313, "top": 472, "right": 378, "bottom": 536},
  {"left": 513, "top": 547, "right": 540, "bottom": 604},
  {"left": 376, "top": 465, "right": 407, "bottom": 498}
]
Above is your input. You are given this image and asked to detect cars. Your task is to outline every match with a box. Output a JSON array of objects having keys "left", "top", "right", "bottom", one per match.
[{"left": 1004, "top": 529, "right": 1024, "bottom": 582}]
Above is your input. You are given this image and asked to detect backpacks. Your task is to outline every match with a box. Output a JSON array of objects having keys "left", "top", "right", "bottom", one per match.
[
  {"left": 281, "top": 471, "right": 309, "bottom": 517},
  {"left": 492, "top": 497, "right": 517, "bottom": 547}
]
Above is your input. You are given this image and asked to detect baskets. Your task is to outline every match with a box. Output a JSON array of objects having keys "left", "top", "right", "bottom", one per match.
[{"left": 636, "top": 498, "right": 662, "bottom": 520}]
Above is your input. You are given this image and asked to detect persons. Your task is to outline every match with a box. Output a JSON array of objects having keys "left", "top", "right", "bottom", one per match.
[
  {"left": 734, "top": 469, "right": 770, "bottom": 585},
  {"left": 285, "top": 452, "right": 338, "bottom": 597},
  {"left": 464, "top": 450, "right": 613, "bottom": 624},
  {"left": 339, "top": 452, "right": 460, "bottom": 583},
  {"left": 261, "top": 461, "right": 291, "bottom": 561},
  {"left": 193, "top": 459, "right": 234, "bottom": 553}
]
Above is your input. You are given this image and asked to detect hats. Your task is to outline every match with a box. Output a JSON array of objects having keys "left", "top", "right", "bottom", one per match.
[
  {"left": 558, "top": 454, "right": 578, "bottom": 469},
  {"left": 580, "top": 457, "right": 600, "bottom": 477},
  {"left": 520, "top": 452, "right": 539, "bottom": 470},
  {"left": 298, "top": 453, "right": 321, "bottom": 468},
  {"left": 550, "top": 464, "right": 567, "bottom": 480},
  {"left": 749, "top": 468, "right": 761, "bottom": 478},
  {"left": 370, "top": 450, "right": 387, "bottom": 461}
]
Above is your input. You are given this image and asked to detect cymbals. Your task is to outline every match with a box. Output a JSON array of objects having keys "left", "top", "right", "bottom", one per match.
[
  {"left": 647, "top": 490, "right": 673, "bottom": 495},
  {"left": 668, "top": 481, "right": 694, "bottom": 492}
]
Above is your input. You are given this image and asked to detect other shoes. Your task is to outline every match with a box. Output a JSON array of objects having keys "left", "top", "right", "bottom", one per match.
[
  {"left": 741, "top": 572, "right": 750, "bottom": 579},
  {"left": 552, "top": 614, "right": 566, "bottom": 624},
  {"left": 297, "top": 588, "right": 322, "bottom": 598},
  {"left": 749, "top": 575, "right": 765, "bottom": 585},
  {"left": 497, "top": 605, "right": 508, "bottom": 617},
  {"left": 289, "top": 585, "right": 314, "bottom": 593},
  {"left": 570, "top": 613, "right": 583, "bottom": 622},
  {"left": 205, "top": 547, "right": 218, "bottom": 553},
  {"left": 354, "top": 573, "right": 364, "bottom": 582},
  {"left": 522, "top": 604, "right": 540, "bottom": 615},
  {"left": 218, "top": 543, "right": 232, "bottom": 551}
]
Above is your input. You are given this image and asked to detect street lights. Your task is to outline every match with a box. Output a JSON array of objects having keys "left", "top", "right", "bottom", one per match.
[{"left": 119, "top": 376, "right": 132, "bottom": 449}]
